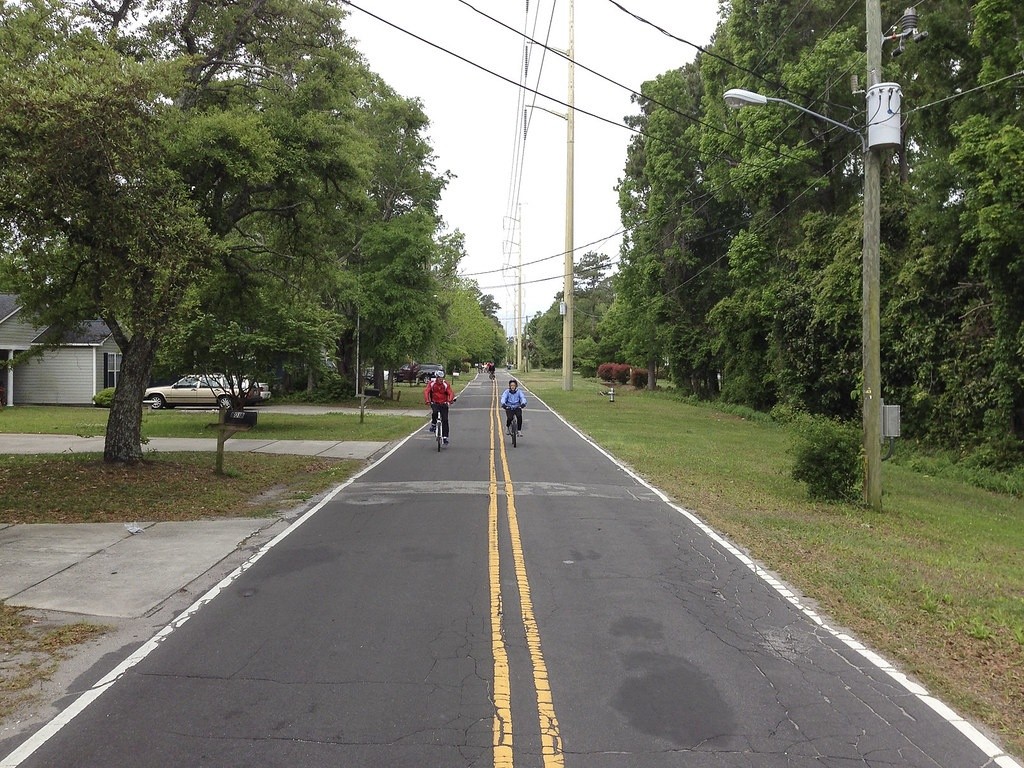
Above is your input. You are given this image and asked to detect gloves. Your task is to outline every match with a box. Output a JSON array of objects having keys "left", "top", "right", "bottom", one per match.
[
  {"left": 521, "top": 403, "right": 526, "bottom": 409},
  {"left": 502, "top": 403, "right": 507, "bottom": 409}
]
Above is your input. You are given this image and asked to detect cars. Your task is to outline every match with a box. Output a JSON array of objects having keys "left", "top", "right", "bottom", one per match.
[
  {"left": 226, "top": 376, "right": 271, "bottom": 402},
  {"left": 394, "top": 363, "right": 445, "bottom": 383},
  {"left": 357, "top": 367, "right": 392, "bottom": 381},
  {"left": 141, "top": 373, "right": 260, "bottom": 410}
]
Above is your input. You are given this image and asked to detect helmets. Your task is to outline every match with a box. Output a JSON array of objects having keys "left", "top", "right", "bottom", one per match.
[
  {"left": 435, "top": 370, "right": 445, "bottom": 378},
  {"left": 509, "top": 379, "right": 518, "bottom": 387}
]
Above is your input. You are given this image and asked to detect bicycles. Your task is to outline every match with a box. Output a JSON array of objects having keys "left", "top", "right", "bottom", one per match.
[
  {"left": 504, "top": 404, "right": 527, "bottom": 448},
  {"left": 426, "top": 401, "right": 456, "bottom": 452}
]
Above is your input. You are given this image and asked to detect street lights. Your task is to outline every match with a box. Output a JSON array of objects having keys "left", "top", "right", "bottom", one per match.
[
  {"left": 720, "top": 86, "right": 885, "bottom": 513},
  {"left": 524, "top": 104, "right": 575, "bottom": 391}
]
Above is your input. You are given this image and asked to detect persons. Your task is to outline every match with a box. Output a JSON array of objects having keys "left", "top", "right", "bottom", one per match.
[
  {"left": 487, "top": 365, "right": 495, "bottom": 379},
  {"left": 501, "top": 380, "right": 528, "bottom": 438},
  {"left": 423, "top": 370, "right": 454, "bottom": 446}
]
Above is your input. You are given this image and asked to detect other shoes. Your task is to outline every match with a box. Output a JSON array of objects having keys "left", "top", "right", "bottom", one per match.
[
  {"left": 444, "top": 437, "right": 449, "bottom": 444},
  {"left": 429, "top": 424, "right": 436, "bottom": 432},
  {"left": 517, "top": 431, "right": 523, "bottom": 437},
  {"left": 506, "top": 426, "right": 510, "bottom": 435}
]
